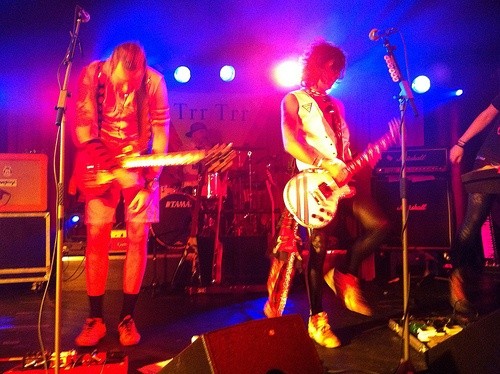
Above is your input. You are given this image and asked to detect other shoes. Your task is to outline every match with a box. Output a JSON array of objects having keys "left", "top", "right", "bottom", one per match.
[
  {"left": 118, "top": 314, "right": 141, "bottom": 347},
  {"left": 74, "top": 317, "right": 107, "bottom": 347}
]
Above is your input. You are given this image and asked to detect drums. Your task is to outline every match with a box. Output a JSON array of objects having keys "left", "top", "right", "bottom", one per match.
[
  {"left": 149, "top": 189, "right": 205, "bottom": 251},
  {"left": 198, "top": 171, "right": 231, "bottom": 200}
]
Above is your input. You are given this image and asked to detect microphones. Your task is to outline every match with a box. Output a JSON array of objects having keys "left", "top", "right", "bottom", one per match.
[
  {"left": 76, "top": 5, "right": 90, "bottom": 23},
  {"left": 369, "top": 27, "right": 399, "bottom": 41}
]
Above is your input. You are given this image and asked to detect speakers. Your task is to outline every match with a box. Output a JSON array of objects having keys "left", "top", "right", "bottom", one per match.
[
  {"left": 370, "top": 175, "right": 454, "bottom": 251},
  {"left": 159, "top": 314, "right": 329, "bottom": 374},
  {"left": 0, "top": 153, "right": 48, "bottom": 213},
  {"left": 423, "top": 307, "right": 500, "bottom": 374}
]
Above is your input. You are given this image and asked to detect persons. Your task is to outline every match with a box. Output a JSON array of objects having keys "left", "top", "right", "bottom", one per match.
[
  {"left": 281, "top": 43, "right": 390, "bottom": 348},
  {"left": 182, "top": 123, "right": 208, "bottom": 189},
  {"left": 449, "top": 92, "right": 500, "bottom": 264},
  {"left": 66, "top": 43, "right": 170, "bottom": 347}
]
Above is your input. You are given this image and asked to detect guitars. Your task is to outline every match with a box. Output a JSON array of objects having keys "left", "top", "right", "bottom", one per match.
[
  {"left": 281, "top": 116, "right": 405, "bottom": 226},
  {"left": 73, "top": 134, "right": 230, "bottom": 198}
]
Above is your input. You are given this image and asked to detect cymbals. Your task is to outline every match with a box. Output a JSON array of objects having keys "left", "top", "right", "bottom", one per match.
[{"left": 231, "top": 145, "right": 267, "bottom": 151}]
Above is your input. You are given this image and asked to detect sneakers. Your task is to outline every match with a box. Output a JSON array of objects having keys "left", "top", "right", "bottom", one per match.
[
  {"left": 324, "top": 268, "right": 374, "bottom": 317},
  {"left": 308, "top": 311, "right": 342, "bottom": 348}
]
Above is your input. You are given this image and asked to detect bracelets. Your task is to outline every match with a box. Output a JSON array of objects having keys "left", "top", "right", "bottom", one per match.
[
  {"left": 456, "top": 140, "right": 466, "bottom": 148},
  {"left": 312, "top": 156, "right": 323, "bottom": 167}
]
